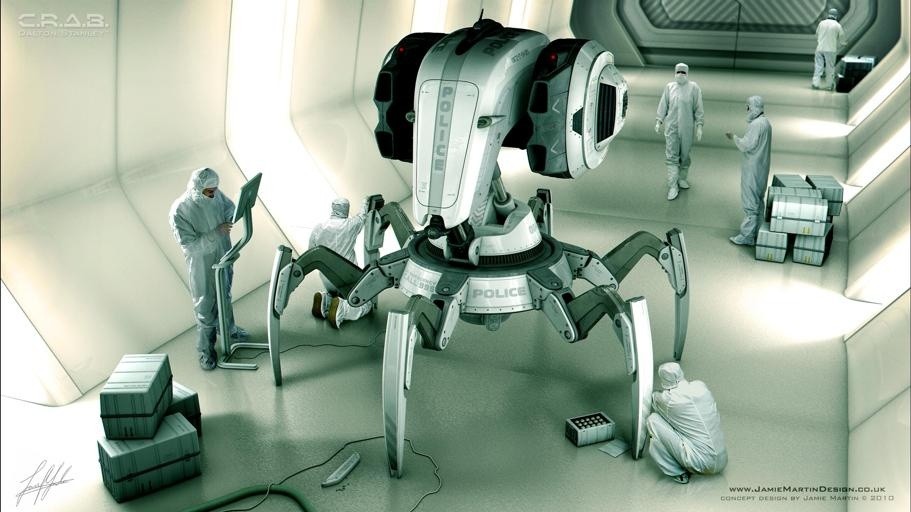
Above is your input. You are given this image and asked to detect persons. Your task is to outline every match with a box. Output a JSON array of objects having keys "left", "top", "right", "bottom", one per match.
[
  {"left": 643, "top": 361, "right": 727, "bottom": 485},
  {"left": 309, "top": 198, "right": 372, "bottom": 330},
  {"left": 725, "top": 96, "right": 772, "bottom": 246},
  {"left": 169, "top": 167, "right": 249, "bottom": 370},
  {"left": 812, "top": 8, "right": 847, "bottom": 91},
  {"left": 654, "top": 63, "right": 705, "bottom": 202}
]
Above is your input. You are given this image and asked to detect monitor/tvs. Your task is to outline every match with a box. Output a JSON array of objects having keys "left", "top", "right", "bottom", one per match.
[{"left": 232, "top": 172, "right": 262, "bottom": 223}]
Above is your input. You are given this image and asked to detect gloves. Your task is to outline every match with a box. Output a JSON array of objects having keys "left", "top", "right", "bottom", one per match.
[
  {"left": 655, "top": 120, "right": 664, "bottom": 136},
  {"left": 697, "top": 125, "right": 704, "bottom": 142}
]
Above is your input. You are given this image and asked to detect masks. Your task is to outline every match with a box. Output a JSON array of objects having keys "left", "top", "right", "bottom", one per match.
[
  {"left": 197, "top": 192, "right": 216, "bottom": 211},
  {"left": 674, "top": 73, "right": 688, "bottom": 83},
  {"left": 746, "top": 111, "right": 754, "bottom": 122}
]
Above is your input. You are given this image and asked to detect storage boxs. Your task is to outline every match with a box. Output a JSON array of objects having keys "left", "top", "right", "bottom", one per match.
[
  {"left": 95, "top": 354, "right": 207, "bottom": 506},
  {"left": 565, "top": 409, "right": 619, "bottom": 447},
  {"left": 755, "top": 169, "right": 845, "bottom": 268}
]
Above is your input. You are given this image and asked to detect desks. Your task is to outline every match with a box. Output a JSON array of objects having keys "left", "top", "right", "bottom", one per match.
[{"left": 835, "top": 53, "right": 877, "bottom": 92}]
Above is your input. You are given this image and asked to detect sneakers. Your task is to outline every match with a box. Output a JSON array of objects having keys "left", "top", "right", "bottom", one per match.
[
  {"left": 667, "top": 184, "right": 681, "bottom": 201},
  {"left": 198, "top": 352, "right": 217, "bottom": 370},
  {"left": 679, "top": 181, "right": 690, "bottom": 189},
  {"left": 670, "top": 474, "right": 690, "bottom": 483},
  {"left": 225, "top": 330, "right": 250, "bottom": 342},
  {"left": 312, "top": 293, "right": 327, "bottom": 319},
  {"left": 330, "top": 297, "right": 346, "bottom": 329},
  {"left": 730, "top": 233, "right": 759, "bottom": 246}
]
